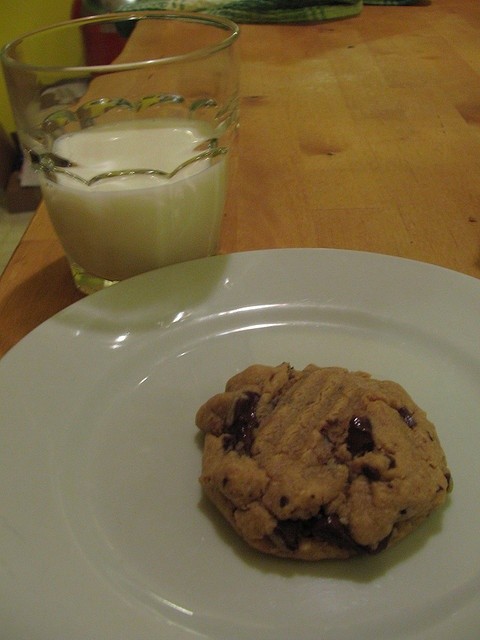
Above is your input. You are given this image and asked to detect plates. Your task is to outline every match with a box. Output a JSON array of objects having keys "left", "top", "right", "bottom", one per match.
[{"left": 0, "top": 247, "right": 480, "bottom": 640}]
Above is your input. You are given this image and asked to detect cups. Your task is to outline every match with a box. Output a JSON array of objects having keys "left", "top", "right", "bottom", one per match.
[{"left": 0, "top": 10, "right": 240, "bottom": 295}]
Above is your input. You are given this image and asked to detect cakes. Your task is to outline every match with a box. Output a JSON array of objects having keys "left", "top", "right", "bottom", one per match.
[{"left": 195, "top": 361, "right": 454, "bottom": 564}]
